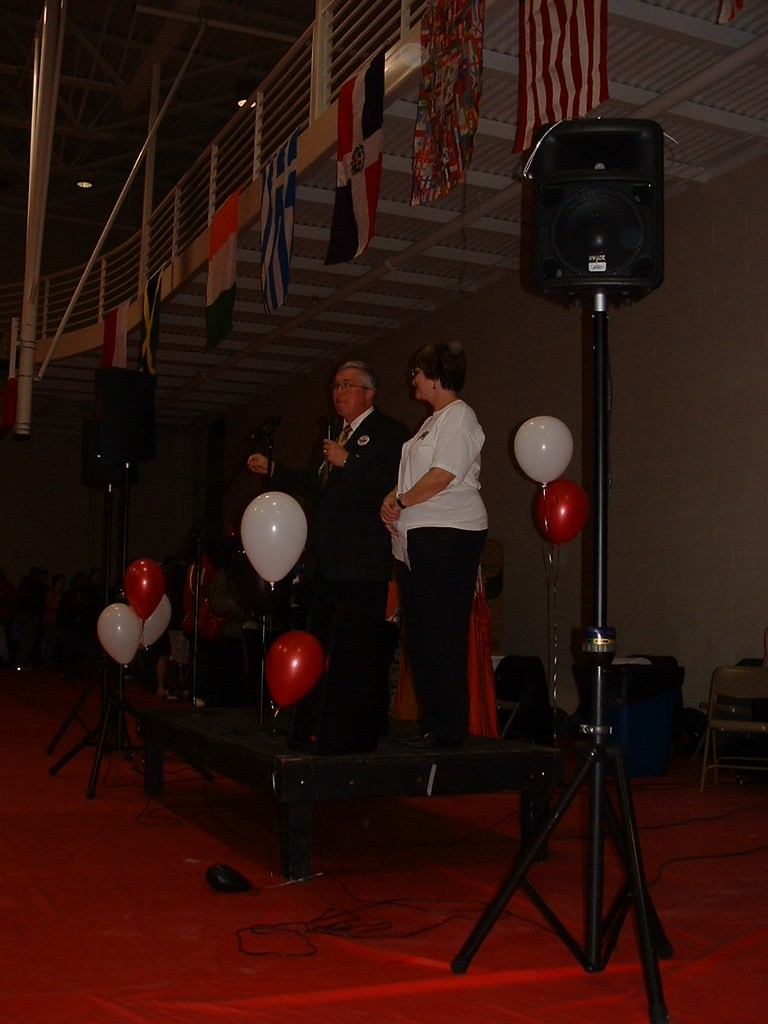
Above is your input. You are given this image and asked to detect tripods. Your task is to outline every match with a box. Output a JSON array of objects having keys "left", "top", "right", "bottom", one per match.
[
  {"left": 45, "top": 468, "right": 138, "bottom": 799},
  {"left": 451, "top": 291, "right": 674, "bottom": 1024}
]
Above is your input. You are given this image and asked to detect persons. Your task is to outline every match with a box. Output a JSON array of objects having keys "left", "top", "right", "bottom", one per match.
[
  {"left": 0, "top": 533, "right": 263, "bottom": 714},
  {"left": 379, "top": 341, "right": 489, "bottom": 749},
  {"left": 248, "top": 361, "right": 411, "bottom": 758}
]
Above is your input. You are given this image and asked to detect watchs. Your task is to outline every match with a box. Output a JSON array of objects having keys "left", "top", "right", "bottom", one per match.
[{"left": 395, "top": 493, "right": 406, "bottom": 509}]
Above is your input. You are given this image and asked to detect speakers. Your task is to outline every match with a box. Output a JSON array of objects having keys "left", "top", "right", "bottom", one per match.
[
  {"left": 530, "top": 118, "right": 665, "bottom": 303},
  {"left": 81, "top": 366, "right": 157, "bottom": 487},
  {"left": 285, "top": 603, "right": 399, "bottom": 756}
]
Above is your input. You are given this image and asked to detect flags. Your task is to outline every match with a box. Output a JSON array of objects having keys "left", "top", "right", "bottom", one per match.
[
  {"left": 136, "top": 265, "right": 163, "bottom": 386},
  {"left": 409, "top": 0, "right": 486, "bottom": 213},
  {"left": 254, "top": 126, "right": 300, "bottom": 318},
  {"left": 94, "top": 300, "right": 131, "bottom": 424},
  {"left": 204, "top": 189, "right": 239, "bottom": 350},
  {"left": 322, "top": 40, "right": 385, "bottom": 268},
  {"left": 509, "top": 0, "right": 609, "bottom": 155},
  {"left": 715, "top": 0, "right": 749, "bottom": 26}
]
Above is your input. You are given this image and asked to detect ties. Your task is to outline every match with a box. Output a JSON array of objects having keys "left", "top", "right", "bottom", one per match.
[{"left": 322, "top": 424, "right": 352, "bottom": 493}]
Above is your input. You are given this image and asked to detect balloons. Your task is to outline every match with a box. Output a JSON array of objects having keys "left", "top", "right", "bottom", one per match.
[
  {"left": 96, "top": 603, "right": 143, "bottom": 669},
  {"left": 239, "top": 491, "right": 310, "bottom": 593},
  {"left": 264, "top": 628, "right": 326, "bottom": 714},
  {"left": 532, "top": 478, "right": 591, "bottom": 548},
  {"left": 122, "top": 556, "right": 166, "bottom": 620},
  {"left": 128, "top": 593, "right": 172, "bottom": 652},
  {"left": 513, "top": 414, "right": 574, "bottom": 489}
]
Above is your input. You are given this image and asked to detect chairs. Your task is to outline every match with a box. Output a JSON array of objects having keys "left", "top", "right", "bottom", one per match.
[
  {"left": 699, "top": 664, "right": 768, "bottom": 795},
  {"left": 490, "top": 654, "right": 555, "bottom": 744}
]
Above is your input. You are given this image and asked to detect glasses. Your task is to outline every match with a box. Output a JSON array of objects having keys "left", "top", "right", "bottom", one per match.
[
  {"left": 332, "top": 381, "right": 369, "bottom": 393},
  {"left": 411, "top": 368, "right": 426, "bottom": 378}
]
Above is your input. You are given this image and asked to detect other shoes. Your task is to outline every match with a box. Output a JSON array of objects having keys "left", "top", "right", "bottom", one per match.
[{"left": 397, "top": 728, "right": 463, "bottom": 750}]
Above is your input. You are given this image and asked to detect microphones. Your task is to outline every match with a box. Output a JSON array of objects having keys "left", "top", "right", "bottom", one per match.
[
  {"left": 325, "top": 415, "right": 334, "bottom": 472},
  {"left": 250, "top": 415, "right": 282, "bottom": 439}
]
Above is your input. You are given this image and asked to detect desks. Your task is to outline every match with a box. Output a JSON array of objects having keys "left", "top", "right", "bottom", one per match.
[{"left": 571, "top": 654, "right": 683, "bottom": 777}]
